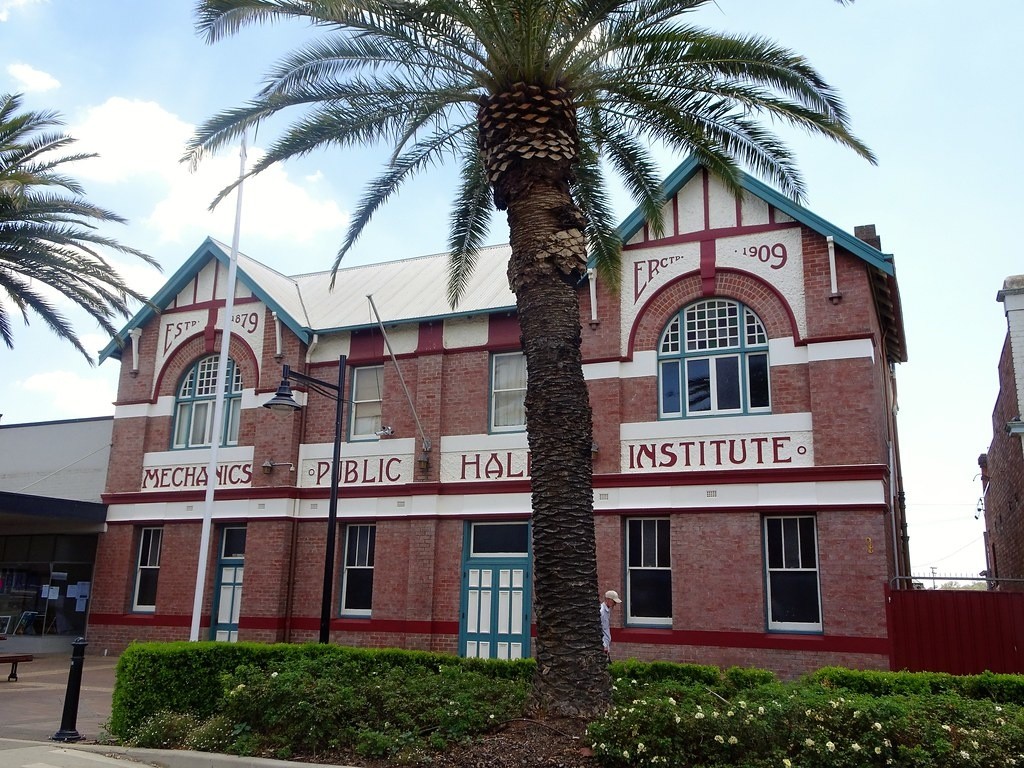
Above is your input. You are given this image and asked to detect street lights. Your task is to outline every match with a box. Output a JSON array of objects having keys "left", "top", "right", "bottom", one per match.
[{"left": 260, "top": 354, "right": 346, "bottom": 645}]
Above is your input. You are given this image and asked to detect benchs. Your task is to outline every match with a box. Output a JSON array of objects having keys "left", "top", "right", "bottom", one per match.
[{"left": 0, "top": 653, "right": 33, "bottom": 681}]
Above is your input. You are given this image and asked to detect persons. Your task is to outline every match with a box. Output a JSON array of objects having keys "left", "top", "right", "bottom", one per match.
[{"left": 599, "top": 589, "right": 623, "bottom": 665}]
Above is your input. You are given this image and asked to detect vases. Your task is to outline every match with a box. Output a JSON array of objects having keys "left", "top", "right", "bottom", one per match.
[{"left": 262, "top": 462, "right": 294, "bottom": 473}]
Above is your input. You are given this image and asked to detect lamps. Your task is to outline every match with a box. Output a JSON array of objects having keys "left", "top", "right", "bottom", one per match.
[
  {"left": 417, "top": 452, "right": 430, "bottom": 469},
  {"left": 591, "top": 444, "right": 599, "bottom": 460}
]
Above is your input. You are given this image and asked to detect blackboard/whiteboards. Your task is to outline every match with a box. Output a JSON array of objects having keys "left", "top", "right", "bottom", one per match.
[{"left": 0, "top": 616, "right": 11, "bottom": 634}]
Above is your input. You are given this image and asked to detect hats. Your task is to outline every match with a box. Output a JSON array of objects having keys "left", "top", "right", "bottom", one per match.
[{"left": 605, "top": 590, "right": 622, "bottom": 603}]
